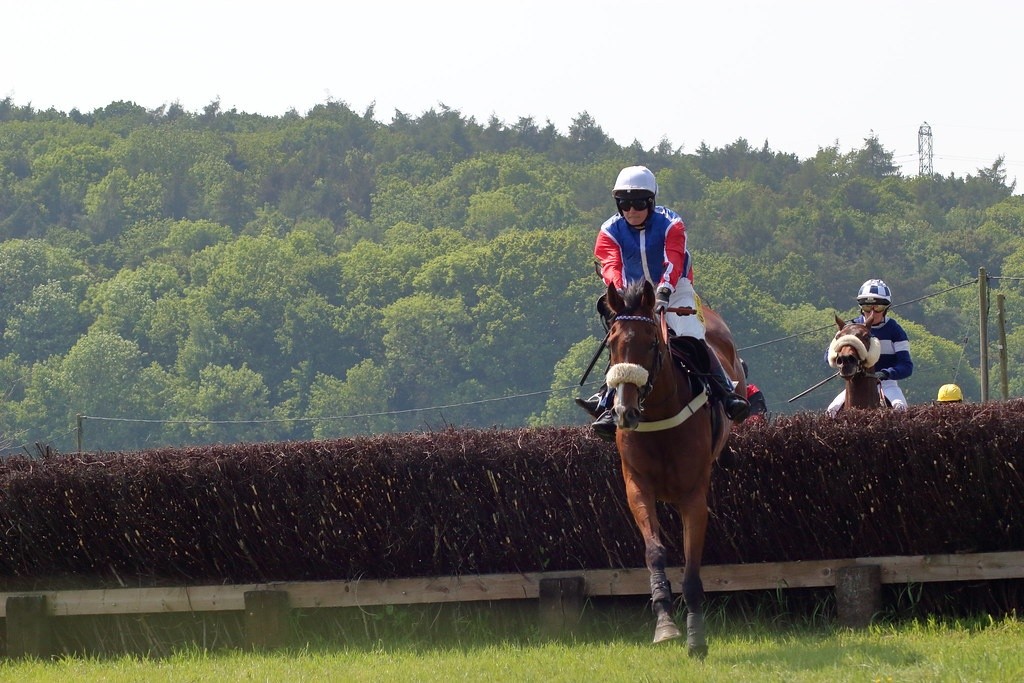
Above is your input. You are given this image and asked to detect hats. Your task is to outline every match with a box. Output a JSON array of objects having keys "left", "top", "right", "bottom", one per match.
[{"left": 857, "top": 279, "right": 892, "bottom": 302}]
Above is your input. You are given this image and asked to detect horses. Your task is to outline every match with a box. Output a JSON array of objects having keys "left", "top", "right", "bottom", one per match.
[
  {"left": 827, "top": 310, "right": 887, "bottom": 410},
  {"left": 604, "top": 275, "right": 748, "bottom": 667}
]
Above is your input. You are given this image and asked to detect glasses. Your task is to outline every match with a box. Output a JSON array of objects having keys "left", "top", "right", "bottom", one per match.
[
  {"left": 862, "top": 305, "right": 887, "bottom": 313},
  {"left": 619, "top": 199, "right": 651, "bottom": 211}
]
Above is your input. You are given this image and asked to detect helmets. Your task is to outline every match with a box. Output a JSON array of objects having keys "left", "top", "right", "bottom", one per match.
[
  {"left": 937, "top": 384, "right": 963, "bottom": 401},
  {"left": 612, "top": 166, "right": 658, "bottom": 197}
]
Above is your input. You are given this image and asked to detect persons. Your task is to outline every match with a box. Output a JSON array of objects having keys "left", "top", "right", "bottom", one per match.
[
  {"left": 936, "top": 384, "right": 963, "bottom": 403},
  {"left": 575, "top": 166, "right": 751, "bottom": 442},
  {"left": 732, "top": 358, "right": 767, "bottom": 424},
  {"left": 824, "top": 279, "right": 913, "bottom": 416}
]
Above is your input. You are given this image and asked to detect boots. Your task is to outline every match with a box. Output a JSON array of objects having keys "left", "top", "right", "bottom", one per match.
[
  {"left": 707, "top": 347, "right": 750, "bottom": 424},
  {"left": 576, "top": 397, "right": 605, "bottom": 420}
]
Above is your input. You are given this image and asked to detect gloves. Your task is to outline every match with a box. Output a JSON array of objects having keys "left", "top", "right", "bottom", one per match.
[
  {"left": 875, "top": 372, "right": 886, "bottom": 384},
  {"left": 653, "top": 287, "right": 671, "bottom": 314}
]
